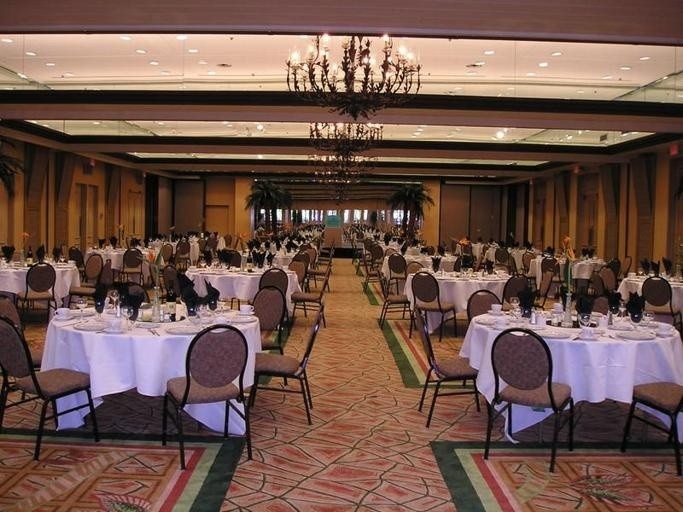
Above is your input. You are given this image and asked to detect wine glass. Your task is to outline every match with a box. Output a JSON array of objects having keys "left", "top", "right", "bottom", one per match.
[
  {"left": 186, "top": 303, "right": 197, "bottom": 324},
  {"left": 629, "top": 312, "right": 643, "bottom": 331},
  {"left": 577, "top": 314, "right": 590, "bottom": 337},
  {"left": 609, "top": 304, "right": 620, "bottom": 328},
  {"left": 109, "top": 290, "right": 119, "bottom": 311},
  {"left": 75, "top": 299, "right": 88, "bottom": 323},
  {"left": 645, "top": 310, "right": 654, "bottom": 326},
  {"left": 207, "top": 300, "right": 217, "bottom": 318}
]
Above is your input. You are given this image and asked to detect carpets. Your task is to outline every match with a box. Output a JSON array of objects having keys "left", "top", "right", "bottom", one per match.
[
  {"left": 429, "top": 439, "right": 682, "bottom": 511},
  {"left": 1, "top": 426, "right": 246, "bottom": 511}
]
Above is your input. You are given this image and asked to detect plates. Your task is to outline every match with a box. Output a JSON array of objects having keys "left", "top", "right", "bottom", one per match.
[
  {"left": 52, "top": 261, "right": 72, "bottom": 269},
  {"left": 236, "top": 310, "right": 255, "bottom": 315},
  {"left": 188, "top": 266, "right": 225, "bottom": 275},
  {"left": 101, "top": 328, "right": 127, "bottom": 333},
  {"left": 416, "top": 267, "right": 455, "bottom": 280},
  {"left": 482, "top": 273, "right": 508, "bottom": 281},
  {"left": 487, "top": 310, "right": 504, "bottom": 315},
  {"left": 616, "top": 332, "right": 655, "bottom": 339},
  {"left": 657, "top": 331, "right": 676, "bottom": 338},
  {"left": 667, "top": 274, "right": 683, "bottom": 286},
  {"left": 51, "top": 314, "right": 74, "bottom": 321},
  {"left": 626, "top": 272, "right": 647, "bottom": 282},
  {"left": 74, "top": 321, "right": 111, "bottom": 331},
  {"left": 475, "top": 316, "right": 510, "bottom": 325},
  {"left": 536, "top": 330, "right": 570, "bottom": 338},
  {"left": 166, "top": 326, "right": 202, "bottom": 334},
  {"left": 228, "top": 316, "right": 257, "bottom": 323}
]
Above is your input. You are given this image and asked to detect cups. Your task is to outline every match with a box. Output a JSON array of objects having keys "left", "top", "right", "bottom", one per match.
[
  {"left": 54, "top": 307, "right": 69, "bottom": 317},
  {"left": 239, "top": 304, "right": 254, "bottom": 312},
  {"left": 216, "top": 317, "right": 231, "bottom": 326},
  {"left": 551, "top": 313, "right": 559, "bottom": 326},
  {"left": 490, "top": 304, "right": 502, "bottom": 312},
  {"left": 659, "top": 324, "right": 674, "bottom": 334}
]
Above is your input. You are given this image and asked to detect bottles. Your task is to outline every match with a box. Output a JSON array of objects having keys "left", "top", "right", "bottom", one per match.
[
  {"left": 530, "top": 308, "right": 536, "bottom": 324},
  {"left": 27, "top": 246, "right": 32, "bottom": 267},
  {"left": 246, "top": 251, "right": 253, "bottom": 271},
  {"left": 162, "top": 299, "right": 169, "bottom": 323},
  {"left": 166, "top": 279, "right": 176, "bottom": 321}
]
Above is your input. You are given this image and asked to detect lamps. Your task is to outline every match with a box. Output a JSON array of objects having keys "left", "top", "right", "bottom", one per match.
[
  {"left": 284, "top": 35, "right": 422, "bottom": 123},
  {"left": 308, "top": 120, "right": 383, "bottom": 203}
]
